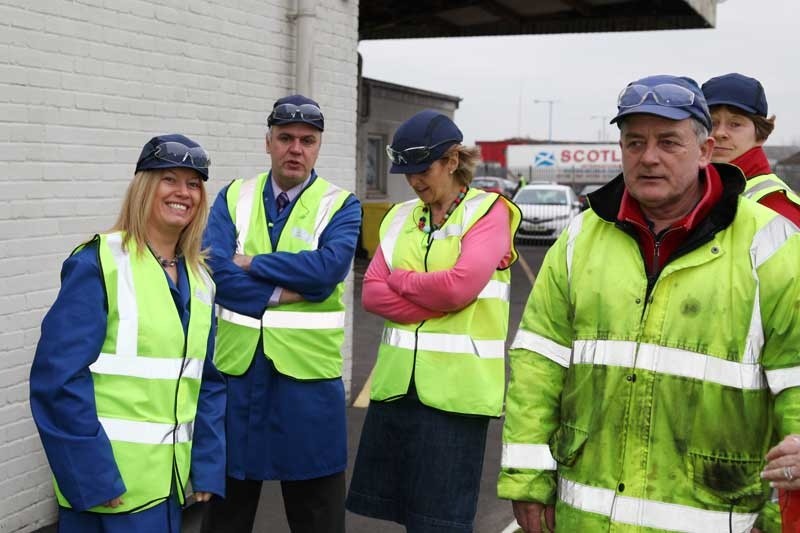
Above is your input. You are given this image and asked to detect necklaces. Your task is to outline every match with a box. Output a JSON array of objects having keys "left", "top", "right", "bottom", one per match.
[
  {"left": 145, "top": 238, "right": 179, "bottom": 267},
  {"left": 418, "top": 185, "right": 467, "bottom": 233}
]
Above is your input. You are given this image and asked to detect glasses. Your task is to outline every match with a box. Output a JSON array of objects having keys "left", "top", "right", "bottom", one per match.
[
  {"left": 267, "top": 104, "right": 324, "bottom": 124},
  {"left": 386, "top": 138, "right": 463, "bottom": 166},
  {"left": 618, "top": 84, "right": 712, "bottom": 122},
  {"left": 135, "top": 142, "right": 211, "bottom": 168}
]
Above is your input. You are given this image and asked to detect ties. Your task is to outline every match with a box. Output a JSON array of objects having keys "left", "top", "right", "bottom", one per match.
[{"left": 276, "top": 192, "right": 290, "bottom": 218}]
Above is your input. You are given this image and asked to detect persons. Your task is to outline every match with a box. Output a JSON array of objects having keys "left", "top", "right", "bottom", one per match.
[
  {"left": 511, "top": 173, "right": 525, "bottom": 200},
  {"left": 498, "top": 71, "right": 800, "bottom": 533},
  {"left": 344, "top": 111, "right": 523, "bottom": 533},
  {"left": 201, "top": 95, "right": 362, "bottom": 533},
  {"left": 29, "top": 135, "right": 226, "bottom": 533}
]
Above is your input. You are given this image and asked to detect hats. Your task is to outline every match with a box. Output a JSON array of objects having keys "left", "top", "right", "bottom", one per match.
[
  {"left": 136, "top": 135, "right": 208, "bottom": 181},
  {"left": 701, "top": 73, "right": 767, "bottom": 118},
  {"left": 610, "top": 75, "right": 712, "bottom": 134},
  {"left": 390, "top": 111, "right": 463, "bottom": 174},
  {"left": 269, "top": 96, "right": 324, "bottom": 132}
]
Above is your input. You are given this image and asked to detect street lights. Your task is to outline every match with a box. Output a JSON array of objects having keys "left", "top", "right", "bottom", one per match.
[{"left": 534, "top": 99, "right": 555, "bottom": 142}]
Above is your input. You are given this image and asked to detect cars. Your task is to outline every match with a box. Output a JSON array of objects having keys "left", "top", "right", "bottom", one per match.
[
  {"left": 511, "top": 184, "right": 585, "bottom": 241},
  {"left": 580, "top": 183, "right": 605, "bottom": 211},
  {"left": 471, "top": 175, "right": 518, "bottom": 201}
]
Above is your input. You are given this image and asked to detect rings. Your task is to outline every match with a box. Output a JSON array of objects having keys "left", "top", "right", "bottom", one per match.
[{"left": 784, "top": 466, "right": 793, "bottom": 481}]
